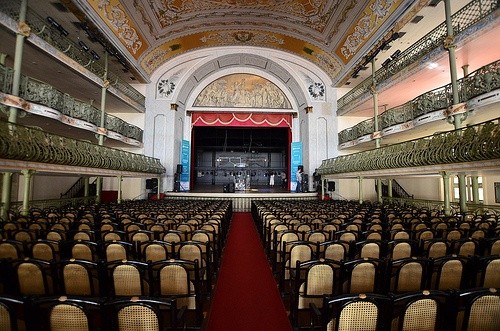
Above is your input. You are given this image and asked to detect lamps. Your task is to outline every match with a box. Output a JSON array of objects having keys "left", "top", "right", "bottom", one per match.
[
  {"left": 79, "top": 21, "right": 129, "bottom": 70},
  {"left": 47, "top": 16, "right": 69, "bottom": 37},
  {"left": 351, "top": 32, "right": 400, "bottom": 78}
]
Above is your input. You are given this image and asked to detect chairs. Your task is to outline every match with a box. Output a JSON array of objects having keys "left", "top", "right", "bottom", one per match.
[
  {"left": 251, "top": 200, "right": 500, "bottom": 331},
  {"left": 0, "top": 199, "right": 232, "bottom": 331}
]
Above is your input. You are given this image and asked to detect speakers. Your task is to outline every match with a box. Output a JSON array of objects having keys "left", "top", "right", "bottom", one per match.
[
  {"left": 328, "top": 181, "right": 335, "bottom": 191},
  {"left": 298, "top": 166, "right": 303, "bottom": 172},
  {"left": 146, "top": 179, "right": 153, "bottom": 189},
  {"left": 223, "top": 182, "right": 235, "bottom": 193}
]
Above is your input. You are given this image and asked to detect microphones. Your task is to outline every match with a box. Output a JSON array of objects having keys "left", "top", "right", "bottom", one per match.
[{"left": 177, "top": 165, "right": 183, "bottom": 173}]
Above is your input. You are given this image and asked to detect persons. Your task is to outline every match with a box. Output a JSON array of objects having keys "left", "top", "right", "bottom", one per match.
[{"left": 270, "top": 168, "right": 320, "bottom": 193}]
